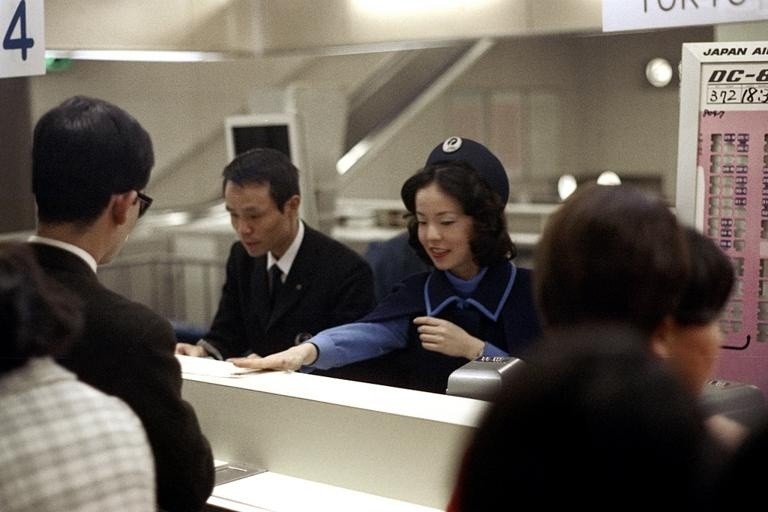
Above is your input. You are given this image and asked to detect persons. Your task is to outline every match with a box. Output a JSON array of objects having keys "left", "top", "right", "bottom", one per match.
[
  {"left": 0, "top": 95, "right": 215, "bottom": 512},
  {"left": 173, "top": 148, "right": 376, "bottom": 383},
  {"left": 225, "top": 135, "right": 544, "bottom": 394},
  {"left": 447, "top": 184, "right": 768, "bottom": 512},
  {"left": 0, "top": 239, "right": 156, "bottom": 512}
]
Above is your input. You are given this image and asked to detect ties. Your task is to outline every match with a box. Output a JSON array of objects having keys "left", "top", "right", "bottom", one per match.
[{"left": 268, "top": 267, "right": 283, "bottom": 310}]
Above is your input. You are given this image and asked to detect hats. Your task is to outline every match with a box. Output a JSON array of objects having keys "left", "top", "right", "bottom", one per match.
[{"left": 422, "top": 135, "right": 509, "bottom": 208}]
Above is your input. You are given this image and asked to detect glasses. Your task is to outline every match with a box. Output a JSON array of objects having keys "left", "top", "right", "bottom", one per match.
[{"left": 136, "top": 193, "right": 154, "bottom": 217}]
